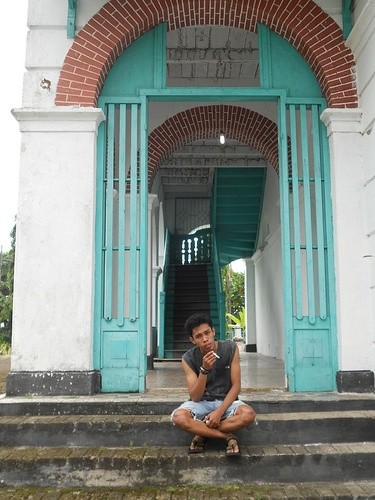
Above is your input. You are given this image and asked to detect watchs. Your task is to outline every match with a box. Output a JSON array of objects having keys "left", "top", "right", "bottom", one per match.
[{"left": 199, "top": 366, "right": 209, "bottom": 374}]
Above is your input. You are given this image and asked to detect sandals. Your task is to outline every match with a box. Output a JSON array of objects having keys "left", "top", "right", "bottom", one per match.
[
  {"left": 225, "top": 433, "right": 242, "bottom": 456},
  {"left": 188, "top": 434, "right": 207, "bottom": 453}
]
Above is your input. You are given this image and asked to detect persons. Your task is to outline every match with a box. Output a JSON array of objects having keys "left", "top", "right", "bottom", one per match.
[{"left": 171, "top": 313, "right": 256, "bottom": 456}]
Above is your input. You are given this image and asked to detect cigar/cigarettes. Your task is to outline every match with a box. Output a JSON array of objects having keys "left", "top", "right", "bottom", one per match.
[{"left": 213, "top": 351, "right": 220, "bottom": 359}]
